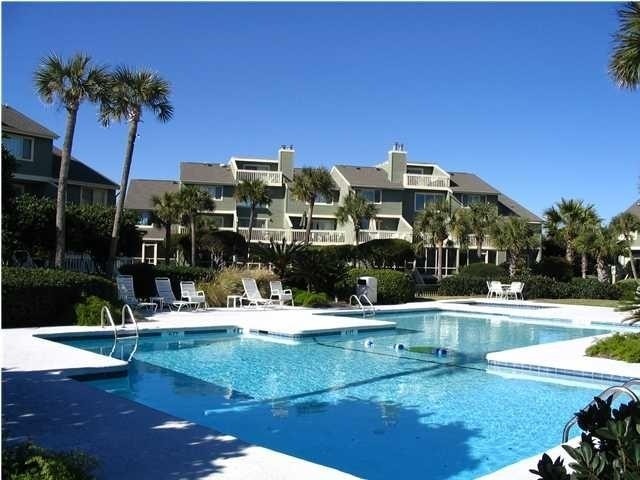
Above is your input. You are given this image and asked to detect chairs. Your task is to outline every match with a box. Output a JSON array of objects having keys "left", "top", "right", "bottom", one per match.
[
  {"left": 486, "top": 281, "right": 525, "bottom": 300},
  {"left": 180, "top": 280, "right": 207, "bottom": 311},
  {"left": 269, "top": 280, "right": 294, "bottom": 309},
  {"left": 239, "top": 278, "right": 274, "bottom": 310},
  {"left": 116, "top": 275, "right": 158, "bottom": 314},
  {"left": 155, "top": 277, "right": 200, "bottom": 312}
]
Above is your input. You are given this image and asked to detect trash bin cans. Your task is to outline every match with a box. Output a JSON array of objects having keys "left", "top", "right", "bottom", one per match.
[{"left": 356, "top": 276, "right": 378, "bottom": 305}]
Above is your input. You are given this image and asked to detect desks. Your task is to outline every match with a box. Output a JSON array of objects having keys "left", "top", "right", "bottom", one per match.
[
  {"left": 226, "top": 295, "right": 242, "bottom": 309},
  {"left": 149, "top": 296, "right": 164, "bottom": 312}
]
